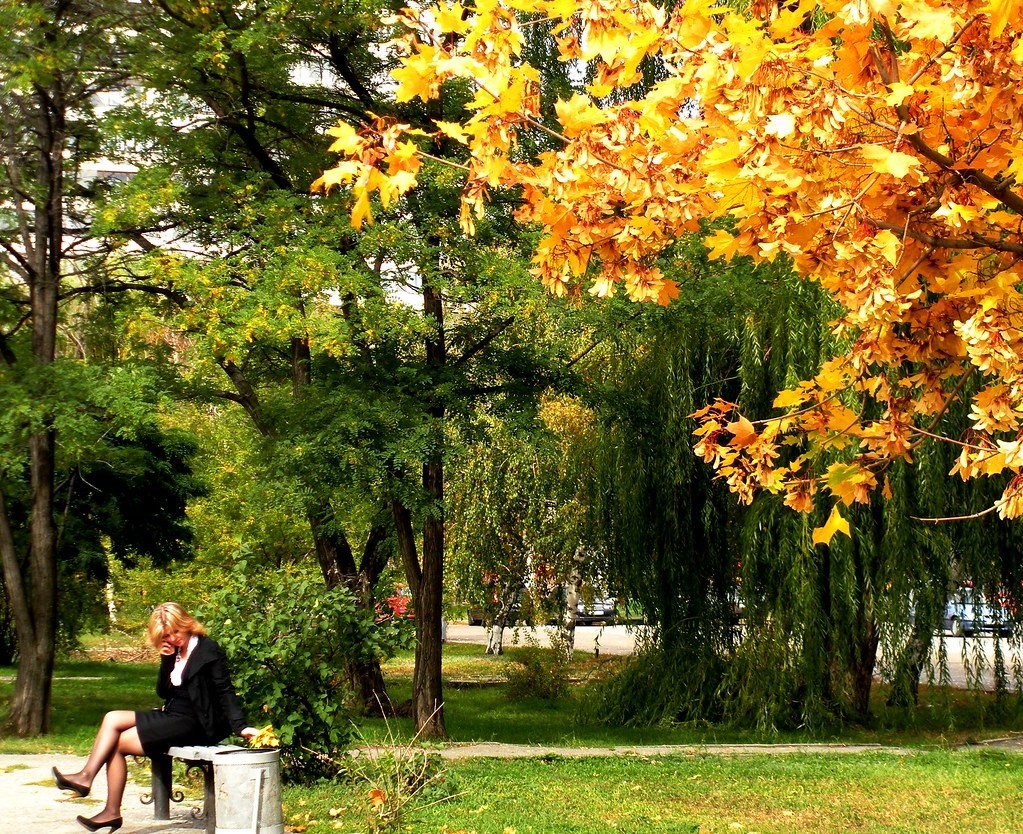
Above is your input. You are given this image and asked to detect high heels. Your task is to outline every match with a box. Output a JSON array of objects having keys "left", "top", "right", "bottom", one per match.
[
  {"left": 52, "top": 767, "right": 91, "bottom": 798},
  {"left": 76, "top": 815, "right": 123, "bottom": 834}
]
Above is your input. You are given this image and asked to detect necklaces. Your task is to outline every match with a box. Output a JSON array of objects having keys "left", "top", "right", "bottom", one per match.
[{"left": 176, "top": 647, "right": 183, "bottom": 662}]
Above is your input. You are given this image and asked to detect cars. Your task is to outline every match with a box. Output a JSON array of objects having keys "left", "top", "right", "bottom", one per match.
[
  {"left": 373, "top": 581, "right": 416, "bottom": 626},
  {"left": 909, "top": 584, "right": 1016, "bottom": 639},
  {"left": 466, "top": 579, "right": 622, "bottom": 627}
]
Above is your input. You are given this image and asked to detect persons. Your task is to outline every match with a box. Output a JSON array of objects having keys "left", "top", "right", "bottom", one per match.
[{"left": 51, "top": 602, "right": 262, "bottom": 834}]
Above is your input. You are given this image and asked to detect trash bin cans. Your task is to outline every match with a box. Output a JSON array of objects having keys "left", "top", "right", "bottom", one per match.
[{"left": 212, "top": 748, "right": 284, "bottom": 834}]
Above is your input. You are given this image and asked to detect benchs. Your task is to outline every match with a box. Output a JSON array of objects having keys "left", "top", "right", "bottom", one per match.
[{"left": 135, "top": 743, "right": 249, "bottom": 834}]
